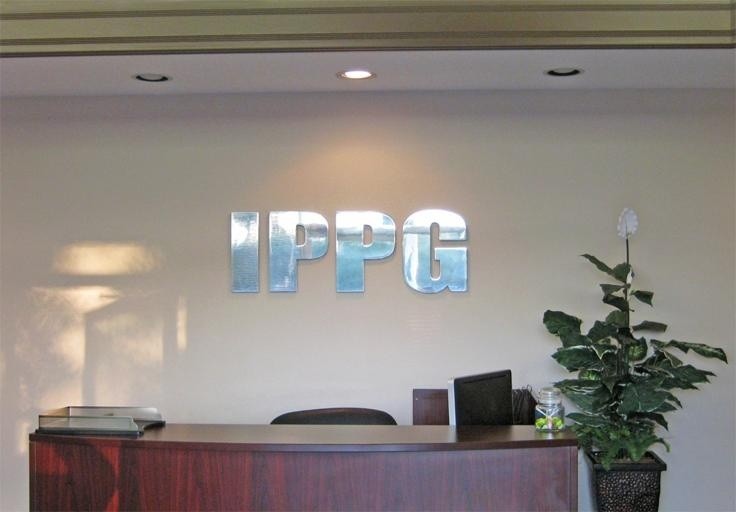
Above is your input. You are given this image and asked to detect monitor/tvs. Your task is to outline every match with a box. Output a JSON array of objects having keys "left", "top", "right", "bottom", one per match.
[{"left": 454, "top": 370, "right": 514, "bottom": 424}]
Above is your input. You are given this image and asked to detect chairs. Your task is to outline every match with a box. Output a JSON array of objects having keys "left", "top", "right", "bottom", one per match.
[{"left": 270, "top": 407, "right": 397, "bottom": 426}]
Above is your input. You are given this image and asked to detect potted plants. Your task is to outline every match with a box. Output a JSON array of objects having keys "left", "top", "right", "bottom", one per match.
[{"left": 543, "top": 208, "right": 730, "bottom": 512}]
